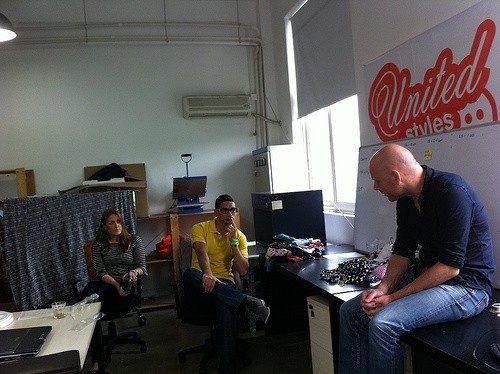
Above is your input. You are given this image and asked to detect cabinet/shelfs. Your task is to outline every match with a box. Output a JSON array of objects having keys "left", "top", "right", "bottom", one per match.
[
  {"left": 136, "top": 209, "right": 241, "bottom": 309},
  {"left": 305, "top": 293, "right": 334, "bottom": 373}
]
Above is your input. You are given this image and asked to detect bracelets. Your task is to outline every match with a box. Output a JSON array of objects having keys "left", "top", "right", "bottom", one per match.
[
  {"left": 230, "top": 240, "right": 239, "bottom": 245},
  {"left": 132, "top": 270, "right": 139, "bottom": 276}
]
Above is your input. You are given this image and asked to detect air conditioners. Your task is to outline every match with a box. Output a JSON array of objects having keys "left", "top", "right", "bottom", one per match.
[{"left": 183, "top": 94, "right": 251, "bottom": 118}]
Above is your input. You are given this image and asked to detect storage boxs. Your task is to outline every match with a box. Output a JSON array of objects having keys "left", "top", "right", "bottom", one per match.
[{"left": 82, "top": 163, "right": 150, "bottom": 218}]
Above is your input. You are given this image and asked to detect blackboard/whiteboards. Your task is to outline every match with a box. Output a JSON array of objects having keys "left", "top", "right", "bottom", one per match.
[{"left": 354, "top": 120, "right": 500, "bottom": 291}]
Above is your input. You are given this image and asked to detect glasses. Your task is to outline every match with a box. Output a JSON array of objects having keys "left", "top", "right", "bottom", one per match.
[{"left": 218, "top": 208, "right": 237, "bottom": 214}]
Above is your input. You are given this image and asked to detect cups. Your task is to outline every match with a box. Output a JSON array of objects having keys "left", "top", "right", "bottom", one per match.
[{"left": 51, "top": 301, "right": 67, "bottom": 319}]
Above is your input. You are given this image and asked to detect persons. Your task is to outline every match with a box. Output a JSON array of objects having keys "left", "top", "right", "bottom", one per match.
[
  {"left": 339, "top": 144, "right": 495, "bottom": 374},
  {"left": 183, "top": 194, "right": 270, "bottom": 374},
  {"left": 82, "top": 210, "right": 146, "bottom": 374}
]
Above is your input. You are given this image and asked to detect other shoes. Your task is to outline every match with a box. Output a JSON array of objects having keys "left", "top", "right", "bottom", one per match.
[
  {"left": 243, "top": 296, "right": 265, "bottom": 309},
  {"left": 87, "top": 361, "right": 102, "bottom": 374}
]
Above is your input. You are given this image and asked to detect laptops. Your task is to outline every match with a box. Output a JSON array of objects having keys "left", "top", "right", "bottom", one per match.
[{"left": 0, "top": 325, "right": 52, "bottom": 362}]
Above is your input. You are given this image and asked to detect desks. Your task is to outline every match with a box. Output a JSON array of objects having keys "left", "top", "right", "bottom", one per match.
[
  {"left": 246, "top": 241, "right": 500, "bottom": 374},
  {"left": 0, "top": 302, "right": 102, "bottom": 374}
]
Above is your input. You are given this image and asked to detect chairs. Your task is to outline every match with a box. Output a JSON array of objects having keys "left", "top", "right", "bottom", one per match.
[
  {"left": 180, "top": 240, "right": 251, "bottom": 374},
  {"left": 83, "top": 241, "right": 147, "bottom": 353}
]
